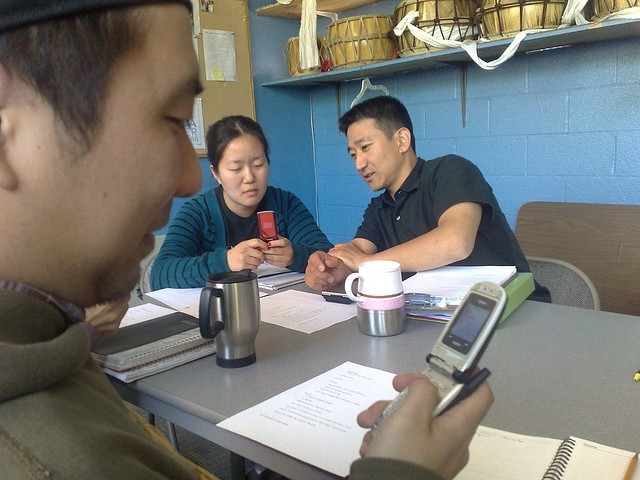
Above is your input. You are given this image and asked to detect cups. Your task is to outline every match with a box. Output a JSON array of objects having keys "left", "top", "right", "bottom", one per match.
[
  {"left": 345, "top": 260, "right": 406, "bottom": 337},
  {"left": 198, "top": 268, "right": 261, "bottom": 368}
]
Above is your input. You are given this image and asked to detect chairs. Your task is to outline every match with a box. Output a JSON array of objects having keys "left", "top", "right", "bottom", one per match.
[{"left": 523, "top": 255, "right": 600, "bottom": 313}]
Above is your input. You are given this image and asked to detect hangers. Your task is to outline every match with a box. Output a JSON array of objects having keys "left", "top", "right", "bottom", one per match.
[{"left": 349, "top": 62, "right": 391, "bottom": 111}]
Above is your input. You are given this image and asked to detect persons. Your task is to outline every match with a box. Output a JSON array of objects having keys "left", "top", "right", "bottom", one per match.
[
  {"left": 148, "top": 115, "right": 335, "bottom": 291},
  {"left": 301, "top": 94, "right": 552, "bottom": 301},
  {"left": 0, "top": 2, "right": 496, "bottom": 480}
]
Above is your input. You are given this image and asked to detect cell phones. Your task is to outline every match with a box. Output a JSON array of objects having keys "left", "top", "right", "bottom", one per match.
[
  {"left": 370, "top": 282, "right": 508, "bottom": 439},
  {"left": 257, "top": 211, "right": 279, "bottom": 251}
]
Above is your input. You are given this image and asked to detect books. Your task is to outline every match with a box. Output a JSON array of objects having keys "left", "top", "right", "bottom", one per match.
[
  {"left": 456, "top": 423, "right": 640, "bottom": 480},
  {"left": 86, "top": 301, "right": 216, "bottom": 384},
  {"left": 252, "top": 263, "right": 305, "bottom": 290}
]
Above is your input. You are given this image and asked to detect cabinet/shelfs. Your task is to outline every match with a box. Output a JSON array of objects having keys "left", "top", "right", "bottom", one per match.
[{"left": 253, "top": 0, "right": 639, "bottom": 88}]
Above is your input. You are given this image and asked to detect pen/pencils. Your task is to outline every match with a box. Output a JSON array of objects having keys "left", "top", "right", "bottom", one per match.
[{"left": 442, "top": 368, "right": 492, "bottom": 411}]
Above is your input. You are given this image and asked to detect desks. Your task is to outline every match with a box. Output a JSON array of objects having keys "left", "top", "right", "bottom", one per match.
[{"left": 88, "top": 235, "right": 639, "bottom": 480}]
